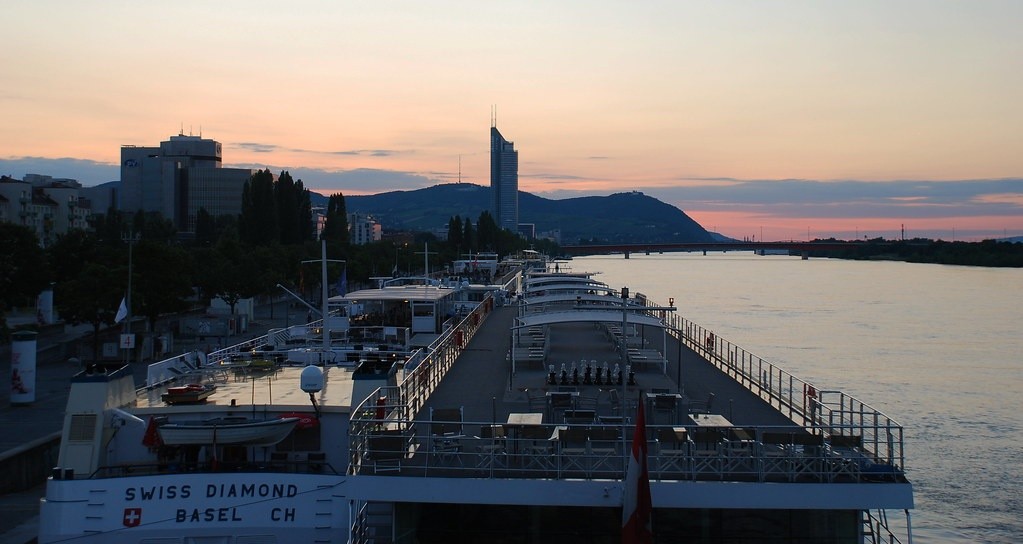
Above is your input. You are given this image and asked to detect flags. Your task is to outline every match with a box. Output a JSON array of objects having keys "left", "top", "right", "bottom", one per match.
[
  {"left": 335, "top": 264, "right": 346, "bottom": 297},
  {"left": 622, "top": 402, "right": 652, "bottom": 544},
  {"left": 298, "top": 268, "right": 304, "bottom": 294},
  {"left": 114, "top": 298, "right": 128, "bottom": 324}
]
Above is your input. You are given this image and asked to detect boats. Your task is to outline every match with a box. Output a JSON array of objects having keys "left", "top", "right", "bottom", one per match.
[
  {"left": 554, "top": 256, "right": 573, "bottom": 260},
  {"left": 36, "top": 260, "right": 915, "bottom": 543}
]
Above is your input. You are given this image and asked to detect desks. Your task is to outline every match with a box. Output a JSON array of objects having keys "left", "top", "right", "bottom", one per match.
[
  {"left": 221, "top": 361, "right": 251, "bottom": 384},
  {"left": 647, "top": 393, "right": 683, "bottom": 423},
  {"left": 546, "top": 391, "right": 580, "bottom": 417},
  {"left": 686, "top": 414, "right": 734, "bottom": 450},
  {"left": 507, "top": 412, "right": 543, "bottom": 465}
]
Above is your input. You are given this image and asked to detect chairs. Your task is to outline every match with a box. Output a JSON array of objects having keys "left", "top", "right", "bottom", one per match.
[
  {"left": 689, "top": 392, "right": 716, "bottom": 414},
  {"left": 605, "top": 322, "right": 649, "bottom": 345},
  {"left": 526, "top": 388, "right": 548, "bottom": 413},
  {"left": 169, "top": 361, "right": 232, "bottom": 385},
  {"left": 547, "top": 409, "right": 624, "bottom": 459},
  {"left": 430, "top": 406, "right": 464, "bottom": 465},
  {"left": 609, "top": 389, "right": 637, "bottom": 417},
  {"left": 671, "top": 421, "right": 754, "bottom": 465},
  {"left": 627, "top": 348, "right": 669, "bottom": 364},
  {"left": 575, "top": 388, "right": 602, "bottom": 411},
  {"left": 506, "top": 325, "right": 551, "bottom": 369},
  {"left": 475, "top": 425, "right": 505, "bottom": 453},
  {"left": 758, "top": 432, "right": 863, "bottom": 480}
]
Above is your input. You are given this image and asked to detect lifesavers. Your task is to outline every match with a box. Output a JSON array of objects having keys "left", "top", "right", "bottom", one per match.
[
  {"left": 807, "top": 382, "right": 817, "bottom": 411},
  {"left": 709, "top": 330, "right": 715, "bottom": 350},
  {"left": 184, "top": 383, "right": 206, "bottom": 391}
]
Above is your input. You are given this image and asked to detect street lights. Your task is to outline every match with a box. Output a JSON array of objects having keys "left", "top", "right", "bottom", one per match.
[
  {"left": 122, "top": 229, "right": 142, "bottom": 363},
  {"left": 396, "top": 242, "right": 408, "bottom": 276}
]
